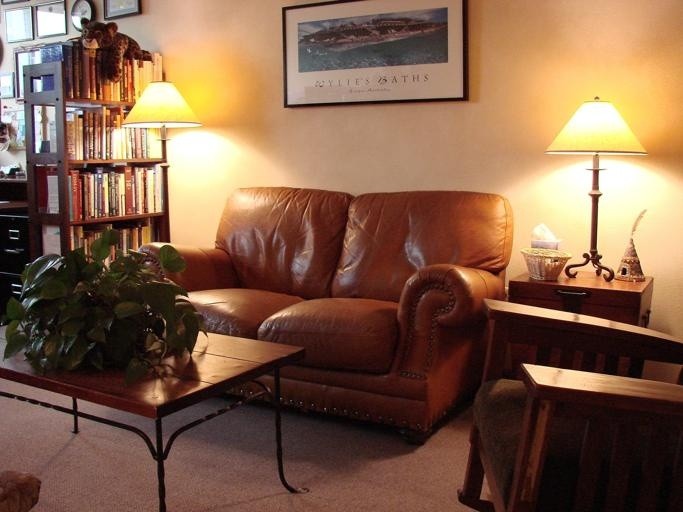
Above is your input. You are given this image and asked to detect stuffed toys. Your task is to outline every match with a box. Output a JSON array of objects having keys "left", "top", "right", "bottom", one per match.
[{"left": 66, "top": 16, "right": 154, "bottom": 83}]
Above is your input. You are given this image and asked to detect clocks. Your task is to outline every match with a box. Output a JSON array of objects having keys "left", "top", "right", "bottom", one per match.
[{"left": 70, "top": 0, "right": 94, "bottom": 32}]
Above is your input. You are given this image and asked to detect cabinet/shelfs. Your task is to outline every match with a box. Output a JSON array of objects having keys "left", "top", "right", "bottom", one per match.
[
  {"left": 22, "top": 61, "right": 172, "bottom": 261},
  {"left": 1, "top": 207, "right": 29, "bottom": 317},
  {"left": 508, "top": 271, "right": 654, "bottom": 373}
]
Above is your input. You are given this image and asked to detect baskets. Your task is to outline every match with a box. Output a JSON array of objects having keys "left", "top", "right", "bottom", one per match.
[{"left": 518, "top": 248, "right": 571, "bottom": 281}]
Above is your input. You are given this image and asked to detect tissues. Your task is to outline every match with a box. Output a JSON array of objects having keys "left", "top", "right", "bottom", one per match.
[{"left": 530, "top": 223, "right": 560, "bottom": 250}]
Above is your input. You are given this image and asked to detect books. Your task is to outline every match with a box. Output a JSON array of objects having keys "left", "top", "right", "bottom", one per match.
[{"left": 42, "top": 41, "right": 164, "bottom": 271}]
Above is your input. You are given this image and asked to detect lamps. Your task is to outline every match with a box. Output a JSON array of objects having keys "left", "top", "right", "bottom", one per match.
[
  {"left": 545, "top": 97, "right": 647, "bottom": 282},
  {"left": 121, "top": 81, "right": 201, "bottom": 244}
]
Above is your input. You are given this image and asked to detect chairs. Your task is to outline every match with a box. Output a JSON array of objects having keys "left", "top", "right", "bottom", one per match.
[{"left": 456, "top": 298, "right": 683, "bottom": 512}]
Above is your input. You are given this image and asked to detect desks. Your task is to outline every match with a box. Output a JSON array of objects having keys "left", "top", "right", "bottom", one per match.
[{"left": 0, "top": 330, "right": 305, "bottom": 512}]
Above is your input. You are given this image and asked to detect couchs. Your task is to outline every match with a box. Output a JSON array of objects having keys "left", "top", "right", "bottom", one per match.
[{"left": 138, "top": 187, "right": 513, "bottom": 445}]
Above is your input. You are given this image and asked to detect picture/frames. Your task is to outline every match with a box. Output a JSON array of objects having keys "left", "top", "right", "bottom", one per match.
[
  {"left": 281, "top": 0, "right": 468, "bottom": 108},
  {"left": 102, "top": 0, "right": 141, "bottom": 20},
  {"left": 0, "top": 0, "right": 66, "bottom": 151}
]
